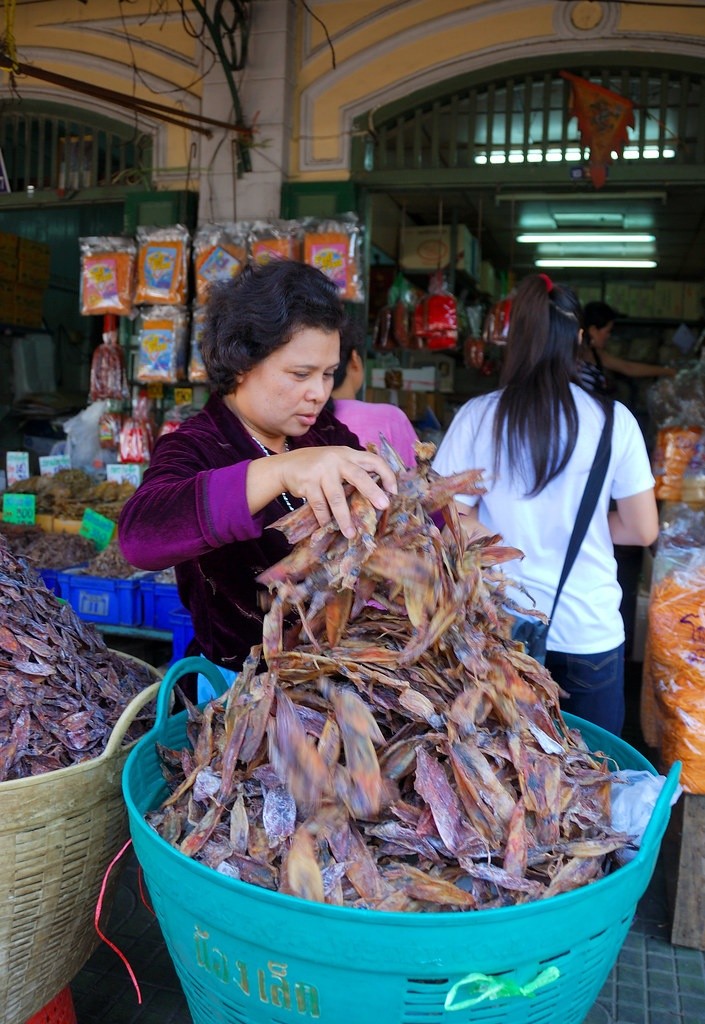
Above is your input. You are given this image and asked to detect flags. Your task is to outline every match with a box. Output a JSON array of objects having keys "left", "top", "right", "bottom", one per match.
[{"left": 568, "top": 76, "right": 636, "bottom": 187}]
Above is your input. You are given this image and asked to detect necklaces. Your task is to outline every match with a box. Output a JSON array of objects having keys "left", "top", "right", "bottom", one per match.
[{"left": 249, "top": 433, "right": 307, "bottom": 512}]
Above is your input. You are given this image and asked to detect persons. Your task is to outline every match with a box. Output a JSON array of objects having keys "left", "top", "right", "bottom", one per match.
[
  {"left": 327, "top": 322, "right": 421, "bottom": 472},
  {"left": 572, "top": 301, "right": 678, "bottom": 396},
  {"left": 420, "top": 270, "right": 661, "bottom": 741},
  {"left": 117, "top": 258, "right": 402, "bottom": 704}
]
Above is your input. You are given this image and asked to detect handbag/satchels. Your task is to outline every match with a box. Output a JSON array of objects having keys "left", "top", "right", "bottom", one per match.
[{"left": 508, "top": 615, "right": 548, "bottom": 668}]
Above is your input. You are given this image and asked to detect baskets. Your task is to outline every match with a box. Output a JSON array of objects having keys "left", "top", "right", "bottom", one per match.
[
  {"left": 122, "top": 655, "right": 681, "bottom": 1024},
  {"left": 0, "top": 648, "right": 174, "bottom": 1024}
]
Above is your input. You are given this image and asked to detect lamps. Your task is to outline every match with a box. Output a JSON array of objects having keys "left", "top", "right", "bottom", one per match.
[{"left": 514, "top": 232, "right": 659, "bottom": 268}]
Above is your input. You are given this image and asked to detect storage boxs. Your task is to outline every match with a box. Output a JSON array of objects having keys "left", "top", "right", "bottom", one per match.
[
  {"left": 371, "top": 366, "right": 436, "bottom": 393},
  {"left": 0, "top": 233, "right": 54, "bottom": 326},
  {"left": 400, "top": 225, "right": 494, "bottom": 295},
  {"left": 36, "top": 556, "right": 195, "bottom": 665}
]
